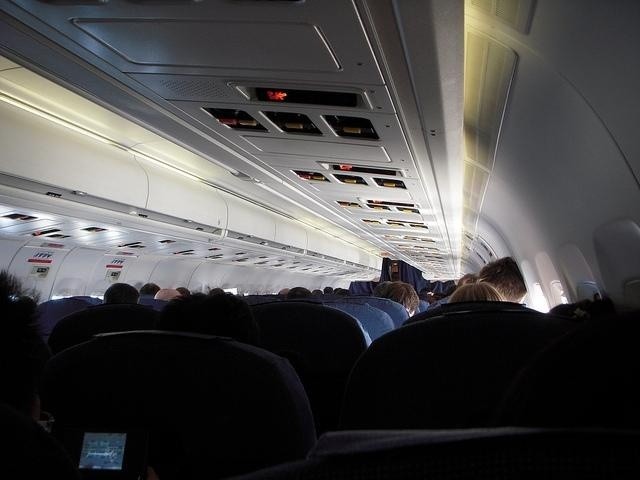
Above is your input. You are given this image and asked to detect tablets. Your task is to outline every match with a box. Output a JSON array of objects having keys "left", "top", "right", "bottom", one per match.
[{"left": 78, "top": 432, "right": 127, "bottom": 471}]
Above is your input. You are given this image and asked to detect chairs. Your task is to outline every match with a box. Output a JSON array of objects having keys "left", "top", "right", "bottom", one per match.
[{"left": 1, "top": 293, "right": 639, "bottom": 480}]
